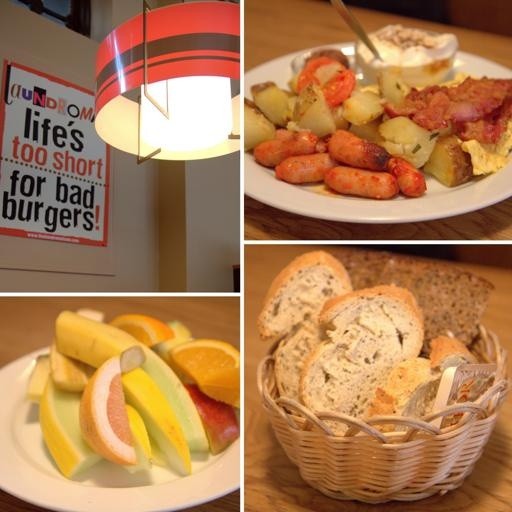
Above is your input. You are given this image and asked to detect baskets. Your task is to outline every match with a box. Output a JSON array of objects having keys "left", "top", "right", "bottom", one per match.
[{"left": 256, "top": 324, "right": 509, "bottom": 503}]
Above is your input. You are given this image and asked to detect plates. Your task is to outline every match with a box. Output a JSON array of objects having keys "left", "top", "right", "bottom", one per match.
[
  {"left": 244, "top": 41, "right": 512, "bottom": 224},
  {"left": 0, "top": 346, "right": 240, "bottom": 512}
]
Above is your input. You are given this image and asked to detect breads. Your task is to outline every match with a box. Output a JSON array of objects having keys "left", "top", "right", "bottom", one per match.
[{"left": 256, "top": 251, "right": 497, "bottom": 433}]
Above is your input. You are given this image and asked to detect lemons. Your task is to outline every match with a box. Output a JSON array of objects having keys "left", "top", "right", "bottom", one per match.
[{"left": 39, "top": 315, "right": 241, "bottom": 479}]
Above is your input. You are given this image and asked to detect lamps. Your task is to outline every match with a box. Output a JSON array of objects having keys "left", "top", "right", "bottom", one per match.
[{"left": 94, "top": 0, "right": 240, "bottom": 161}]
